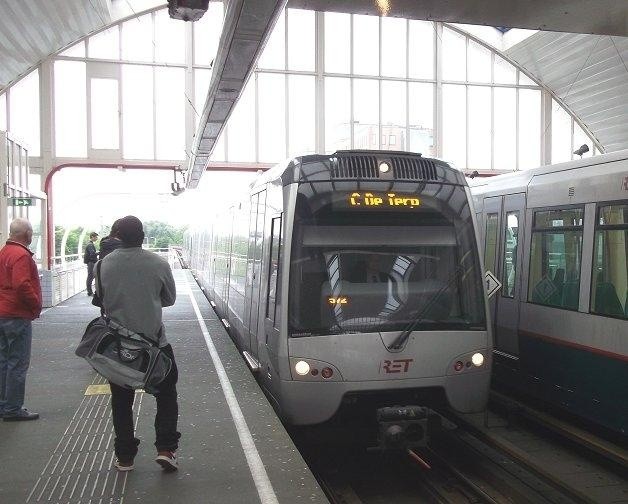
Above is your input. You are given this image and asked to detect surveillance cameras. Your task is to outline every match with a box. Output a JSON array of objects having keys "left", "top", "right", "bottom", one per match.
[{"left": 172, "top": 186, "right": 185, "bottom": 197}]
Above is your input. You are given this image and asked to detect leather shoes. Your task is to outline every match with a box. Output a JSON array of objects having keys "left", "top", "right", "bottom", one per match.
[{"left": 0, "top": 407, "right": 39, "bottom": 421}]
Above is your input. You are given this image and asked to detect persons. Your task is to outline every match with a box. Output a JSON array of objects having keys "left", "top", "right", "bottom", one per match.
[
  {"left": 348, "top": 249, "right": 394, "bottom": 317},
  {"left": 83, "top": 232, "right": 100, "bottom": 296},
  {"left": 92, "top": 213, "right": 182, "bottom": 473},
  {"left": 0, "top": 216, "right": 43, "bottom": 420},
  {"left": 92, "top": 218, "right": 122, "bottom": 317}
]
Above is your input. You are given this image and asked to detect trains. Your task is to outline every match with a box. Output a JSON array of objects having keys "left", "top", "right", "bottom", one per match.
[
  {"left": 182, "top": 149, "right": 494, "bottom": 453},
  {"left": 468, "top": 149, "right": 628, "bottom": 439}
]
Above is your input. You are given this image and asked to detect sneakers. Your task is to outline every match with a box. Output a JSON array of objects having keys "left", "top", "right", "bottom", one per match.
[
  {"left": 155, "top": 450, "right": 178, "bottom": 471},
  {"left": 114, "top": 457, "right": 135, "bottom": 471}
]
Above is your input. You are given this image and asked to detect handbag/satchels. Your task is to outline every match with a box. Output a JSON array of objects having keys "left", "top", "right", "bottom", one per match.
[{"left": 75, "top": 316, "right": 179, "bottom": 394}]
